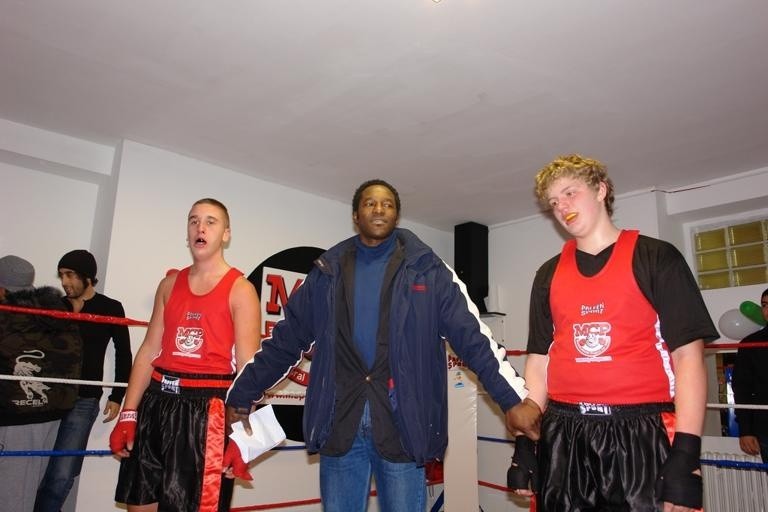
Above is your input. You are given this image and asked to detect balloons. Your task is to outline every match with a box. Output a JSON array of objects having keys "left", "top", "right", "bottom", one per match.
[{"left": 719, "top": 301, "right": 767, "bottom": 340}]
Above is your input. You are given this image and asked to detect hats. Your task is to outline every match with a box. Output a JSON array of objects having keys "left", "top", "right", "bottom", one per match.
[
  {"left": 0, "top": 255, "right": 35, "bottom": 293},
  {"left": 58, "top": 250, "right": 97, "bottom": 277}
]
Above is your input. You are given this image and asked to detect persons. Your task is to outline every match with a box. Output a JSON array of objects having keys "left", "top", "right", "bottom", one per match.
[
  {"left": 731, "top": 289, "right": 768, "bottom": 464},
  {"left": 507, "top": 154, "right": 720, "bottom": 512},
  {"left": 109, "top": 199, "right": 261, "bottom": 511},
  {"left": 217, "top": 434, "right": 254, "bottom": 511},
  {"left": 225, "top": 180, "right": 541, "bottom": 512},
  {"left": 0, "top": 250, "right": 132, "bottom": 512}
]
